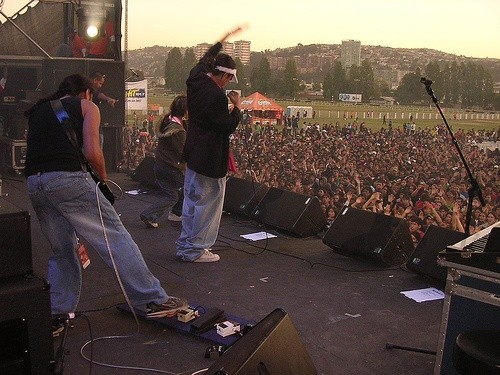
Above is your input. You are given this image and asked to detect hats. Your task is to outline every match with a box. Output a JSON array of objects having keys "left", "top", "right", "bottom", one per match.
[{"left": 215, "top": 65, "right": 238, "bottom": 83}]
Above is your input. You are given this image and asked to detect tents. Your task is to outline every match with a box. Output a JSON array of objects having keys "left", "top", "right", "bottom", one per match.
[{"left": 229, "top": 92, "right": 284, "bottom": 125}]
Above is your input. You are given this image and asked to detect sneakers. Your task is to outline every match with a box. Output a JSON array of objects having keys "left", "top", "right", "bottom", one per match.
[
  {"left": 51, "top": 323, "right": 65, "bottom": 334},
  {"left": 193, "top": 249, "right": 220, "bottom": 263},
  {"left": 168, "top": 211, "right": 182, "bottom": 221},
  {"left": 141, "top": 216, "right": 158, "bottom": 227},
  {"left": 145, "top": 298, "right": 188, "bottom": 320}
]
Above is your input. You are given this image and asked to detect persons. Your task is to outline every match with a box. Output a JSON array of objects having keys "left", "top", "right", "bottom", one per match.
[
  {"left": 174, "top": 27, "right": 245, "bottom": 262},
  {"left": 69, "top": 28, "right": 87, "bottom": 58},
  {"left": 140, "top": 95, "right": 187, "bottom": 227},
  {"left": 87, "top": 10, "right": 119, "bottom": 59},
  {"left": 24, "top": 76, "right": 189, "bottom": 336},
  {"left": 88, "top": 72, "right": 119, "bottom": 152},
  {"left": 122, "top": 109, "right": 500, "bottom": 243}
]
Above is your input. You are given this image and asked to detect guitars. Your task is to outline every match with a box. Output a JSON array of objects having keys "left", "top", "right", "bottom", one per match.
[
  {"left": 88, "top": 165, "right": 116, "bottom": 205},
  {"left": 178, "top": 163, "right": 186, "bottom": 176}
]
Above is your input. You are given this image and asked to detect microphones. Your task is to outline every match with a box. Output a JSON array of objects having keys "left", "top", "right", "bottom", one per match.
[{"left": 231, "top": 91, "right": 235, "bottom": 96}]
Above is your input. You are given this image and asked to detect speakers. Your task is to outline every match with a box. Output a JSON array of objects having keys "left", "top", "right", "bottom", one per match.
[
  {"left": 251, "top": 186, "right": 327, "bottom": 237},
  {"left": 0, "top": 274, "right": 55, "bottom": 375},
  {"left": 130, "top": 156, "right": 162, "bottom": 190},
  {"left": 322, "top": 205, "right": 416, "bottom": 266},
  {"left": 406, "top": 223, "right": 469, "bottom": 282},
  {"left": 222, "top": 175, "right": 269, "bottom": 218},
  {"left": 203, "top": 307, "right": 318, "bottom": 374}
]
